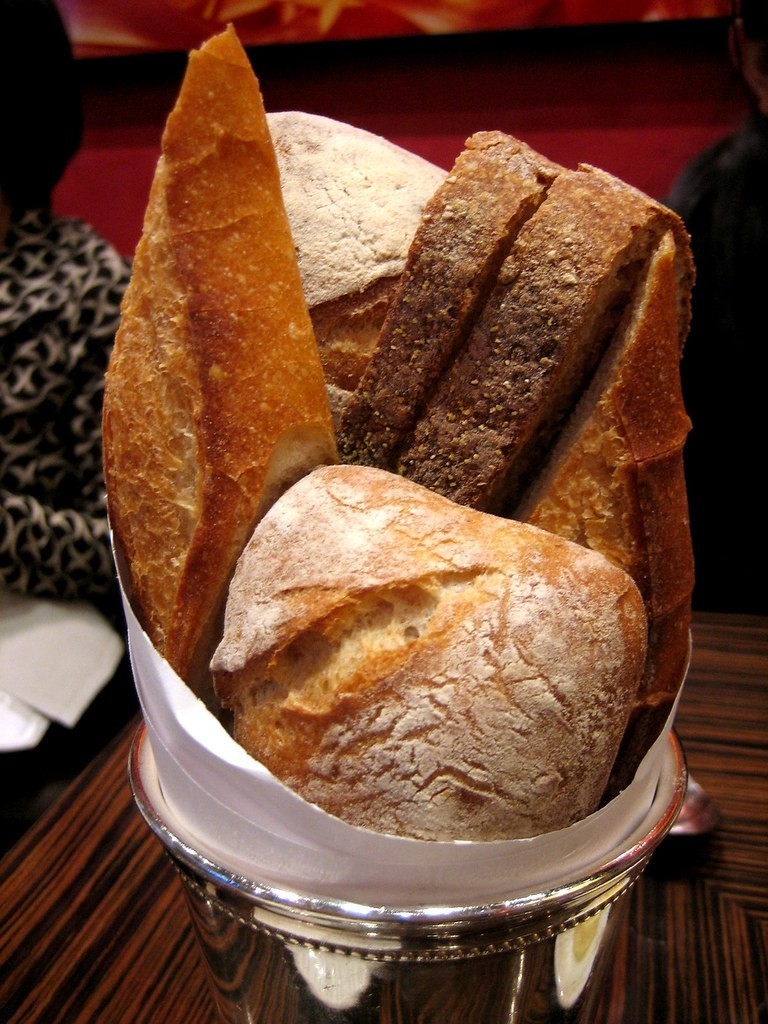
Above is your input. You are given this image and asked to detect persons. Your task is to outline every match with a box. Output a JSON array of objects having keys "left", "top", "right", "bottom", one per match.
[
  {"left": 659, "top": 0, "right": 768, "bottom": 613},
  {"left": 0, "top": 0, "right": 145, "bottom": 836}
]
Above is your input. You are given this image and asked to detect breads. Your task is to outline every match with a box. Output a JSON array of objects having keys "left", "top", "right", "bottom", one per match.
[
  {"left": 208, "top": 463, "right": 647, "bottom": 841},
  {"left": 513, "top": 227, "right": 695, "bottom": 806},
  {"left": 397, "top": 164, "right": 694, "bottom": 520},
  {"left": 335, "top": 129, "right": 572, "bottom": 474},
  {"left": 263, "top": 111, "right": 450, "bottom": 439},
  {"left": 103, "top": 21, "right": 340, "bottom": 692}
]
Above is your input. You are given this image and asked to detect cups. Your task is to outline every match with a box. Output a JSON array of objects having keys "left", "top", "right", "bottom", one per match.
[{"left": 126, "top": 725, "right": 689, "bottom": 1024}]
